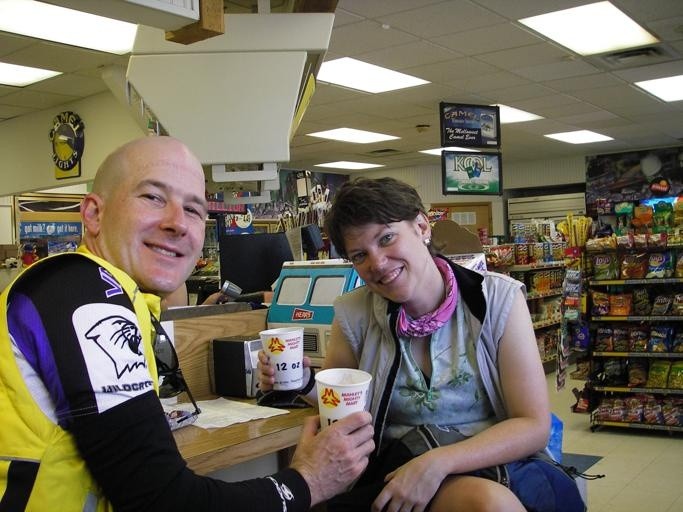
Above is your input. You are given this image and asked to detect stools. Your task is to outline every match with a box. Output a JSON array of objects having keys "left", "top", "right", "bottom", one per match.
[{"left": 560, "top": 445, "right": 605, "bottom": 510}]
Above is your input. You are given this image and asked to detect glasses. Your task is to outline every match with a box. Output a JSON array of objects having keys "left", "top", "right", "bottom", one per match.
[{"left": 150, "top": 308, "right": 201, "bottom": 423}]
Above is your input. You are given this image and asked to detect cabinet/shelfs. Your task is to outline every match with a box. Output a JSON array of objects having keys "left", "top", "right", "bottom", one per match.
[{"left": 1, "top": 189, "right": 683, "bottom": 442}]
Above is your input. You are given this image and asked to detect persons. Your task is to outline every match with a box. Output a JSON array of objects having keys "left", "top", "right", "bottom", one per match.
[
  {"left": 0, "top": 135, "right": 375, "bottom": 512},
  {"left": 256, "top": 177, "right": 587, "bottom": 512},
  {"left": 21, "top": 244, "right": 34, "bottom": 265}
]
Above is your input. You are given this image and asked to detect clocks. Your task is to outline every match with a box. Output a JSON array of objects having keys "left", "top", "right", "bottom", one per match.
[{"left": 47, "top": 110, "right": 86, "bottom": 180}]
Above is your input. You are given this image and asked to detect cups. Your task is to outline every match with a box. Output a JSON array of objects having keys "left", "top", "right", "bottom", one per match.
[
  {"left": 218, "top": 280, "right": 243, "bottom": 300},
  {"left": 258, "top": 326, "right": 305, "bottom": 392},
  {"left": 314, "top": 367, "right": 373, "bottom": 431}
]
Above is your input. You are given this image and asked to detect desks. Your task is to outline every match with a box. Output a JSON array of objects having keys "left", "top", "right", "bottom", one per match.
[{"left": 154, "top": 392, "right": 320, "bottom": 473}]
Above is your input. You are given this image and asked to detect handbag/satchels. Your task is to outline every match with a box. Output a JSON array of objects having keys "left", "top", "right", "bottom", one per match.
[{"left": 368, "top": 424, "right": 604, "bottom": 512}]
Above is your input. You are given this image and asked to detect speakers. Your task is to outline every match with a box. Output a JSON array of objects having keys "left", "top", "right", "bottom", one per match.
[{"left": 212, "top": 335, "right": 265, "bottom": 399}]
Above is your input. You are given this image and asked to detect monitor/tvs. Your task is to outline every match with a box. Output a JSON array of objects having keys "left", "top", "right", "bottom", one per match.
[{"left": 218, "top": 232, "right": 295, "bottom": 303}]
